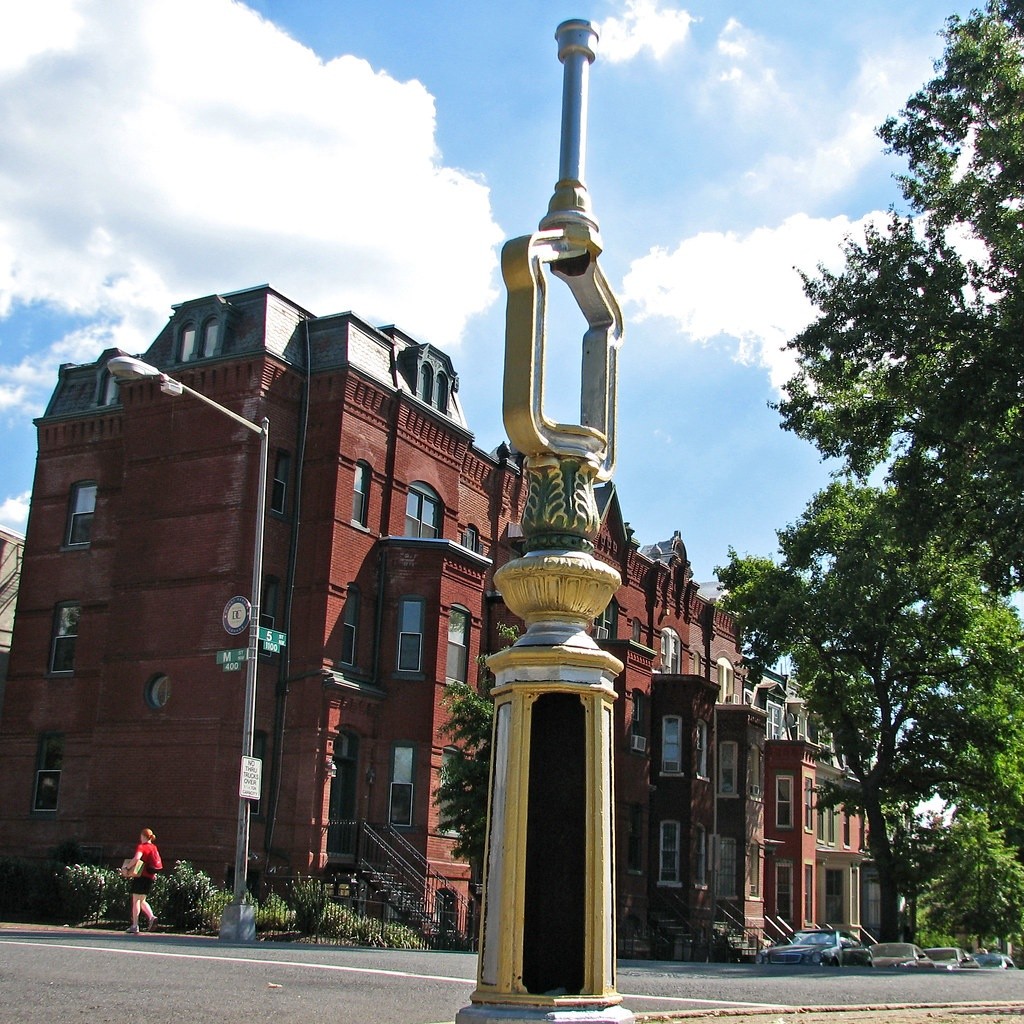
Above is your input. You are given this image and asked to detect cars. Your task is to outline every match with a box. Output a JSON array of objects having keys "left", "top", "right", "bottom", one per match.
[
  {"left": 756, "top": 930, "right": 874, "bottom": 969},
  {"left": 970, "top": 948, "right": 1015, "bottom": 970},
  {"left": 867, "top": 943, "right": 936, "bottom": 969},
  {"left": 921, "top": 948, "right": 980, "bottom": 970}
]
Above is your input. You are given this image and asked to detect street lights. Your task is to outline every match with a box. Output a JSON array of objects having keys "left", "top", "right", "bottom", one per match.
[{"left": 107, "top": 356, "right": 270, "bottom": 945}]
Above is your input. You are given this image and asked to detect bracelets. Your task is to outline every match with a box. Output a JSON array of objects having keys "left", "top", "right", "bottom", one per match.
[{"left": 123, "top": 867, "right": 129, "bottom": 871}]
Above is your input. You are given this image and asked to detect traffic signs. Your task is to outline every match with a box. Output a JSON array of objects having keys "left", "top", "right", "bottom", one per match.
[
  {"left": 217, "top": 648, "right": 249, "bottom": 664},
  {"left": 258, "top": 627, "right": 286, "bottom": 646}
]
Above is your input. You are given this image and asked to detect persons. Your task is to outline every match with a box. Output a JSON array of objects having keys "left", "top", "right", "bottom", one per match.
[{"left": 122, "top": 829, "right": 158, "bottom": 933}]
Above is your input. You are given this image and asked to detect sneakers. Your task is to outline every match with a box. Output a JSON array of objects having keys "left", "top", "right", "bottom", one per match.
[
  {"left": 126, "top": 925, "right": 139, "bottom": 933},
  {"left": 146, "top": 916, "right": 158, "bottom": 931}
]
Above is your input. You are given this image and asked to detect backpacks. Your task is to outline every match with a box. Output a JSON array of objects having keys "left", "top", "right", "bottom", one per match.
[{"left": 145, "top": 849, "right": 162, "bottom": 873}]
